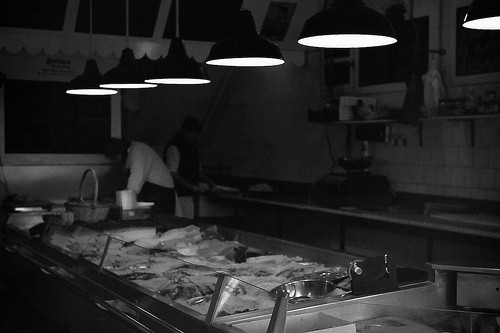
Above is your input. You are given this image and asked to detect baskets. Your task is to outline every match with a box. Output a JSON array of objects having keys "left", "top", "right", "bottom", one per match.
[{"left": 64, "top": 168, "right": 113, "bottom": 223}]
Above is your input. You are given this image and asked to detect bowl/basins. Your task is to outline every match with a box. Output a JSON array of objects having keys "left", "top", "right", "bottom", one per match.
[{"left": 269, "top": 278, "right": 335, "bottom": 305}]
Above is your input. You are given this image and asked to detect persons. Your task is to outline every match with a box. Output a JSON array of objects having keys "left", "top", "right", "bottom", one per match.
[
  {"left": 166, "top": 116, "right": 217, "bottom": 221},
  {"left": 105, "top": 137, "right": 176, "bottom": 217}
]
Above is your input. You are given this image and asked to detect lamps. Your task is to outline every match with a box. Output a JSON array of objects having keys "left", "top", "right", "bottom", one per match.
[
  {"left": 297, "top": 0, "right": 397, "bottom": 49},
  {"left": 65, "top": 0, "right": 120, "bottom": 94},
  {"left": 462, "top": 0, "right": 500, "bottom": 32},
  {"left": 206, "top": 0, "right": 286, "bottom": 67},
  {"left": 143, "top": 0, "right": 212, "bottom": 85},
  {"left": 99, "top": 0, "right": 163, "bottom": 89}
]
